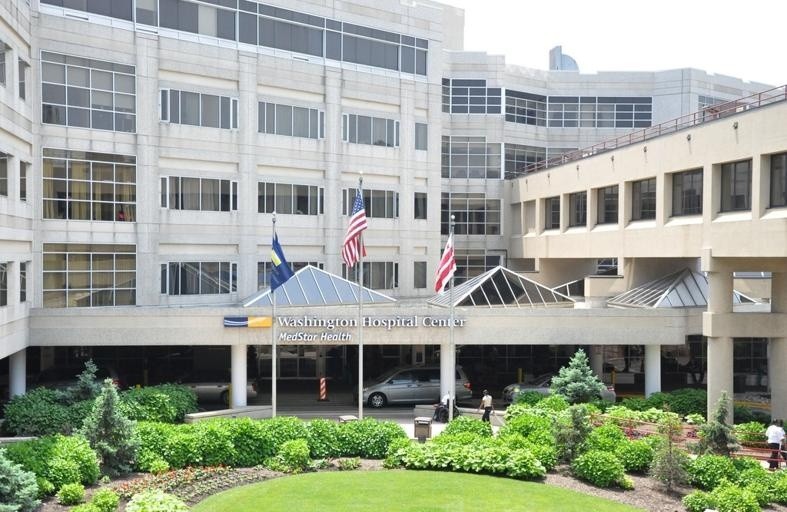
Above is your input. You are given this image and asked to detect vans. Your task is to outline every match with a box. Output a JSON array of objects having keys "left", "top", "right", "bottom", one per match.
[{"left": 353, "top": 364, "right": 473, "bottom": 408}]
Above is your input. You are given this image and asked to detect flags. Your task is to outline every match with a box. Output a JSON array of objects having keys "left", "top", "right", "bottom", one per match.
[
  {"left": 341, "top": 178, "right": 368, "bottom": 270},
  {"left": 269, "top": 226, "right": 295, "bottom": 293},
  {"left": 434, "top": 224, "right": 457, "bottom": 294}
]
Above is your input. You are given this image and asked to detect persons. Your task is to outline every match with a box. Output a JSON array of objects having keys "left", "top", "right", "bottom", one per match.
[
  {"left": 765, "top": 418, "right": 787, "bottom": 469},
  {"left": 478, "top": 389, "right": 494, "bottom": 425},
  {"left": 117, "top": 206, "right": 125, "bottom": 221},
  {"left": 432, "top": 390, "right": 450, "bottom": 421}
]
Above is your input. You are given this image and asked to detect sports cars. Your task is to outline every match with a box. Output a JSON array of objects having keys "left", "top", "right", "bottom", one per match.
[{"left": 159, "top": 367, "right": 260, "bottom": 407}]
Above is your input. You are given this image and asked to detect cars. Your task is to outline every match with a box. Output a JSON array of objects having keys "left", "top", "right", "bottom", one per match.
[
  {"left": 501, "top": 372, "right": 616, "bottom": 406},
  {"left": 36, "top": 362, "right": 131, "bottom": 402}
]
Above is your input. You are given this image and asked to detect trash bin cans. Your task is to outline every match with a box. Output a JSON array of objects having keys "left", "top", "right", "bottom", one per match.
[{"left": 338, "top": 415, "right": 358, "bottom": 424}]
